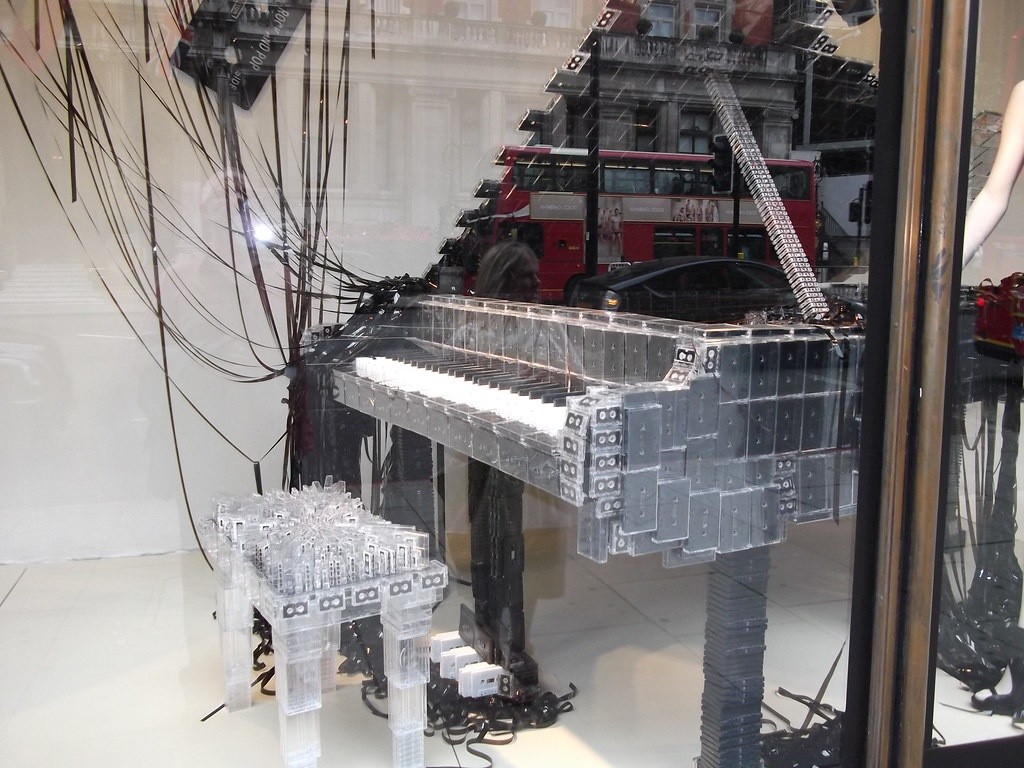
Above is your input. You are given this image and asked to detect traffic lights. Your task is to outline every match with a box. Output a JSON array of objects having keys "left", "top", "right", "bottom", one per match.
[{"left": 861, "top": 180, "right": 871, "bottom": 224}]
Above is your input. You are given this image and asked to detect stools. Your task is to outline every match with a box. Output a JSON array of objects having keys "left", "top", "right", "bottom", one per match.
[{"left": 214, "top": 473, "right": 449, "bottom": 768}]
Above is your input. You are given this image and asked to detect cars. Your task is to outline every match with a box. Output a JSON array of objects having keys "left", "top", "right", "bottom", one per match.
[{"left": 570, "top": 253, "right": 867, "bottom": 326}]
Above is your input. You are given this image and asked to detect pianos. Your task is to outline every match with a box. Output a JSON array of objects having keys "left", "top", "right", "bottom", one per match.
[{"left": 297, "top": 270, "right": 872, "bottom": 768}]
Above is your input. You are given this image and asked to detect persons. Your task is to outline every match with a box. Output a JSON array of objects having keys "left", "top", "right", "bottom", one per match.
[
  {"left": 437, "top": 243, "right": 588, "bottom": 660},
  {"left": 786, "top": 175, "right": 805, "bottom": 198},
  {"left": 674, "top": 199, "right": 719, "bottom": 222},
  {"left": 960, "top": 78, "right": 1024, "bottom": 272},
  {"left": 596, "top": 206, "right": 622, "bottom": 257}
]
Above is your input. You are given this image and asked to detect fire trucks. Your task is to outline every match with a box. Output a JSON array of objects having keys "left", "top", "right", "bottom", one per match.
[{"left": 974, "top": 270, "right": 1024, "bottom": 365}]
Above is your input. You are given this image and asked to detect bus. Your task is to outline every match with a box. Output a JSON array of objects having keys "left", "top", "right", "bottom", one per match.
[{"left": 471, "top": 143, "right": 817, "bottom": 304}]
